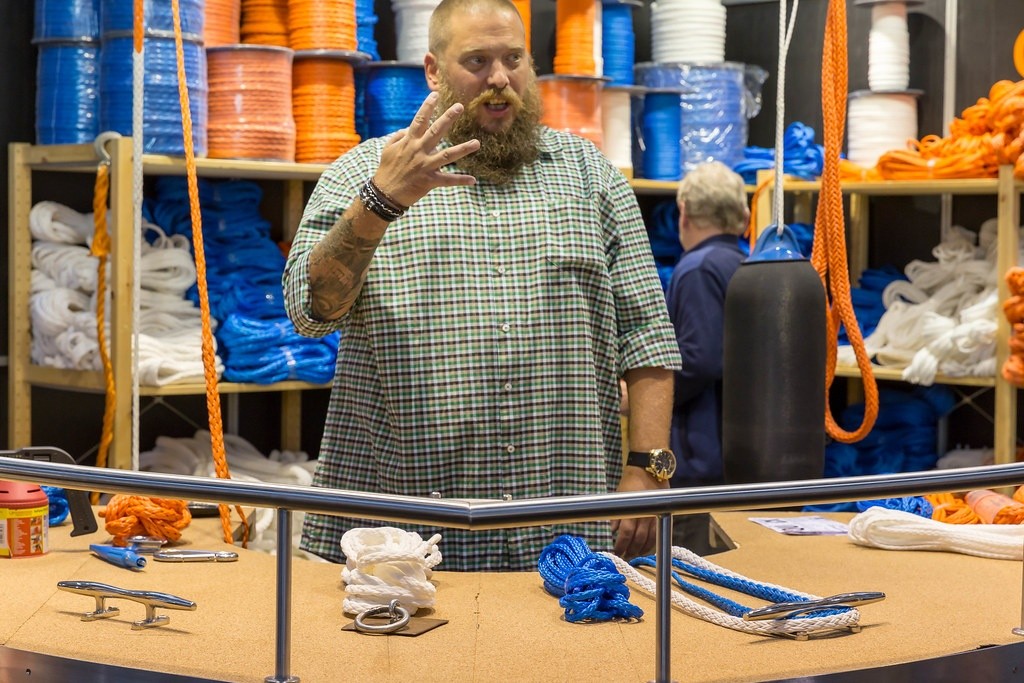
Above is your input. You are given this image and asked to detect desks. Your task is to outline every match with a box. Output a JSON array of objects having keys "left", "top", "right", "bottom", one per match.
[{"left": 0, "top": 505, "right": 1024, "bottom": 683}]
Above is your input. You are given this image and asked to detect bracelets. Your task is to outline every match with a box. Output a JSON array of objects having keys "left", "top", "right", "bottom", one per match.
[{"left": 359, "top": 176, "right": 409, "bottom": 222}]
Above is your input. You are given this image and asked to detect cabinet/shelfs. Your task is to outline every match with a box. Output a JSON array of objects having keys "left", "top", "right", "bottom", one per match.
[{"left": 6, "top": 136, "right": 1024, "bottom": 471}]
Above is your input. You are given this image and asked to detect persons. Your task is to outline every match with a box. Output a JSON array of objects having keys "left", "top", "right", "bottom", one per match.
[
  {"left": 620, "top": 161, "right": 749, "bottom": 556},
  {"left": 281, "top": 0, "right": 682, "bottom": 573}
]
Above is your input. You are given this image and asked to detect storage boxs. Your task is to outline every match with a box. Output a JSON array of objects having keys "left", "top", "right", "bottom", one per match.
[{"left": 0, "top": 479, "right": 49, "bottom": 559}]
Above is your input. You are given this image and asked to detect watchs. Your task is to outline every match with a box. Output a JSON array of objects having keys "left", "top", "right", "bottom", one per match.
[{"left": 626, "top": 448, "right": 677, "bottom": 482}]
